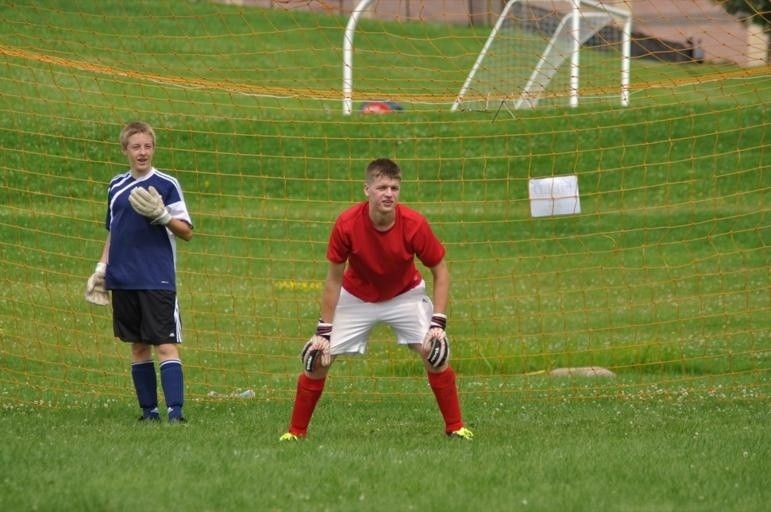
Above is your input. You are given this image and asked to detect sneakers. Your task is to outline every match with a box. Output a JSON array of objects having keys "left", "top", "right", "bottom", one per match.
[
  {"left": 449, "top": 428, "right": 474, "bottom": 442},
  {"left": 279, "top": 433, "right": 303, "bottom": 443}
]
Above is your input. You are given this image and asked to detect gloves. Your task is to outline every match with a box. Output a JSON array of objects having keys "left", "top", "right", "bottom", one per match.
[
  {"left": 128, "top": 186, "right": 171, "bottom": 225},
  {"left": 298, "top": 322, "right": 334, "bottom": 372},
  {"left": 84, "top": 272, "right": 110, "bottom": 306},
  {"left": 423, "top": 313, "right": 450, "bottom": 369}
]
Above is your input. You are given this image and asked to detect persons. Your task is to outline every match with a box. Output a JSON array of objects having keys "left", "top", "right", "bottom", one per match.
[
  {"left": 85, "top": 122, "right": 194, "bottom": 423},
  {"left": 278, "top": 159, "right": 474, "bottom": 443}
]
